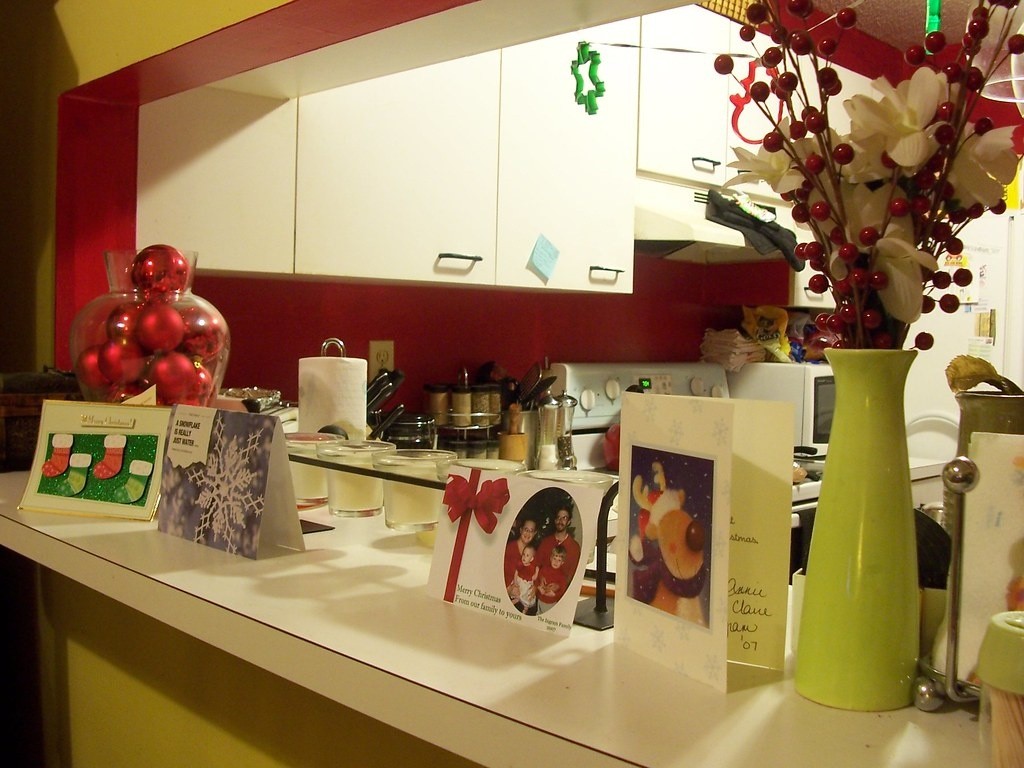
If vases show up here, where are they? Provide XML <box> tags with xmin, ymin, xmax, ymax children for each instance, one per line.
<box><xmin>795</xmin><ymin>345</ymin><xmax>924</xmax><ymax>710</ymax></box>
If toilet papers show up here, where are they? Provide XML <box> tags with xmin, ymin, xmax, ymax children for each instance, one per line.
<box><xmin>297</xmin><ymin>357</ymin><xmax>368</xmax><ymax>441</ymax></box>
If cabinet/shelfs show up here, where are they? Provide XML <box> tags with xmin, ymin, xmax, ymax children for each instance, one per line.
<box><xmin>134</xmin><ymin>2</ymin><xmax>840</xmax><ymax>306</ymax></box>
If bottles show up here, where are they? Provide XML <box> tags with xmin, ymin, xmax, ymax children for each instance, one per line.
<box><xmin>554</xmin><ymin>388</ymin><xmax>578</xmax><ymax>469</ymax></box>
<box><xmin>974</xmin><ymin>608</ymin><xmax>1022</xmax><ymax>768</ymax></box>
<box><xmin>533</xmin><ymin>388</ymin><xmax>563</xmax><ymax>470</ymax></box>
<box><xmin>69</xmin><ymin>250</ymin><xmax>231</xmax><ymax>409</ymax></box>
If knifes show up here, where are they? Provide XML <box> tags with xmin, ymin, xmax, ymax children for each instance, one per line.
<box><xmin>523</xmin><ymin>375</ymin><xmax>557</xmax><ymax>404</ymax></box>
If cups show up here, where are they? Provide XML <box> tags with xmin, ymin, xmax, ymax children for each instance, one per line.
<box><xmin>316</xmin><ymin>441</ymin><xmax>396</xmax><ymax>517</ymax></box>
<box><xmin>435</xmin><ymin>458</ymin><xmax>525</xmax><ymax>483</ymax></box>
<box><xmin>517</xmin><ymin>470</ymin><xmax>614</xmax><ymax>563</ymax></box>
<box><xmin>372</xmin><ymin>450</ymin><xmax>458</xmax><ymax>531</ymax></box>
<box><xmin>285</xmin><ymin>432</ymin><xmax>345</xmax><ymax>507</ymax></box>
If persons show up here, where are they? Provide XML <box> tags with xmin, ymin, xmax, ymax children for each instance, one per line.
<box><xmin>504</xmin><ymin>505</ymin><xmax>580</xmax><ymax>615</ymax></box>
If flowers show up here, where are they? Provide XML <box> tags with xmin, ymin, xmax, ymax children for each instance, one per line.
<box><xmin>714</xmin><ymin>0</ymin><xmax>1024</xmax><ymax>356</ymax></box>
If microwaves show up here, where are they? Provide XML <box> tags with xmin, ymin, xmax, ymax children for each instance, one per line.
<box><xmin>725</xmin><ymin>363</ymin><xmax>835</xmax><ymax>458</ymax></box>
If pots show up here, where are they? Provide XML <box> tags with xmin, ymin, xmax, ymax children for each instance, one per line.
<box><xmin>384</xmin><ymin>413</ymin><xmax>437</xmax><ymax>449</ymax></box>
